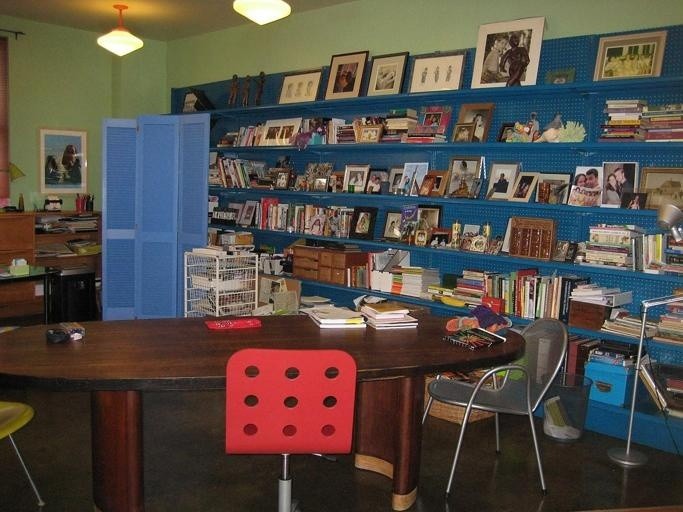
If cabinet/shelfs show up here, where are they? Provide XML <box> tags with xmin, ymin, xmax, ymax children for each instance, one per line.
<box><xmin>0</xmin><ymin>209</ymin><xmax>103</xmax><ymax>320</ymax></box>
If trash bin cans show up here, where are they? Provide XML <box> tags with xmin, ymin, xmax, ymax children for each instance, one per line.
<box><xmin>541</xmin><ymin>372</ymin><xmax>592</xmax><ymax>443</ymax></box>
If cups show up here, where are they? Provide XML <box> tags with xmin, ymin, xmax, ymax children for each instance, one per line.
<box><xmin>76</xmin><ymin>199</ymin><xmax>85</xmax><ymax>210</ymax></box>
<box><xmin>86</xmin><ymin>201</ymin><xmax>94</xmax><ymax>211</ymax></box>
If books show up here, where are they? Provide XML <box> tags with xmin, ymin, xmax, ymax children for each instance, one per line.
<box><xmin>34</xmin><ymin>213</ymin><xmax>103</xmax><ymax>298</ymax></box>
<box><xmin>187</xmin><ymin>99</ymin><xmax>682</xmax><ymax>420</ymax></box>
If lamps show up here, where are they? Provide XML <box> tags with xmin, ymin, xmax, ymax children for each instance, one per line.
<box><xmin>96</xmin><ymin>4</ymin><xmax>145</xmax><ymax>57</ymax></box>
<box><xmin>232</xmin><ymin>0</ymin><xmax>293</xmax><ymax>26</ymax></box>
<box><xmin>0</xmin><ymin>161</ymin><xmax>26</xmax><ymax>214</ymax></box>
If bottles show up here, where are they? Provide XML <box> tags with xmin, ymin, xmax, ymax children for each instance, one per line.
<box><xmin>18</xmin><ymin>193</ymin><xmax>24</xmax><ymax>211</ymax></box>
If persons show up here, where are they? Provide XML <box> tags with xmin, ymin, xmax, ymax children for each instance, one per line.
<box><xmin>602</xmin><ymin>51</ymin><xmax>654</xmax><ymax>77</ymax></box>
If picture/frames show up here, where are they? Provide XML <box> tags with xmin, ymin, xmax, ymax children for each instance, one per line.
<box><xmin>468</xmin><ymin>14</ymin><xmax>547</xmax><ymax>88</ymax></box>
<box><xmin>38</xmin><ymin>128</ymin><xmax>88</xmax><ymax>195</ymax></box>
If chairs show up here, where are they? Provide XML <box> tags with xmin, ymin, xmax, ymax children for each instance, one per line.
<box><xmin>421</xmin><ymin>317</ymin><xmax>569</xmax><ymax>501</ymax></box>
<box><xmin>222</xmin><ymin>348</ymin><xmax>359</xmax><ymax>512</ymax></box>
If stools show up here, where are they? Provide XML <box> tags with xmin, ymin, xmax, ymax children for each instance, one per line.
<box><xmin>0</xmin><ymin>400</ymin><xmax>49</xmax><ymax>507</ymax></box>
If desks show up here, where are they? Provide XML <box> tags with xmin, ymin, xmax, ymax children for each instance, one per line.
<box><xmin>0</xmin><ymin>310</ymin><xmax>529</xmax><ymax>512</ymax></box>
<box><xmin>1</xmin><ymin>264</ymin><xmax>63</xmax><ymax>325</ymax></box>
